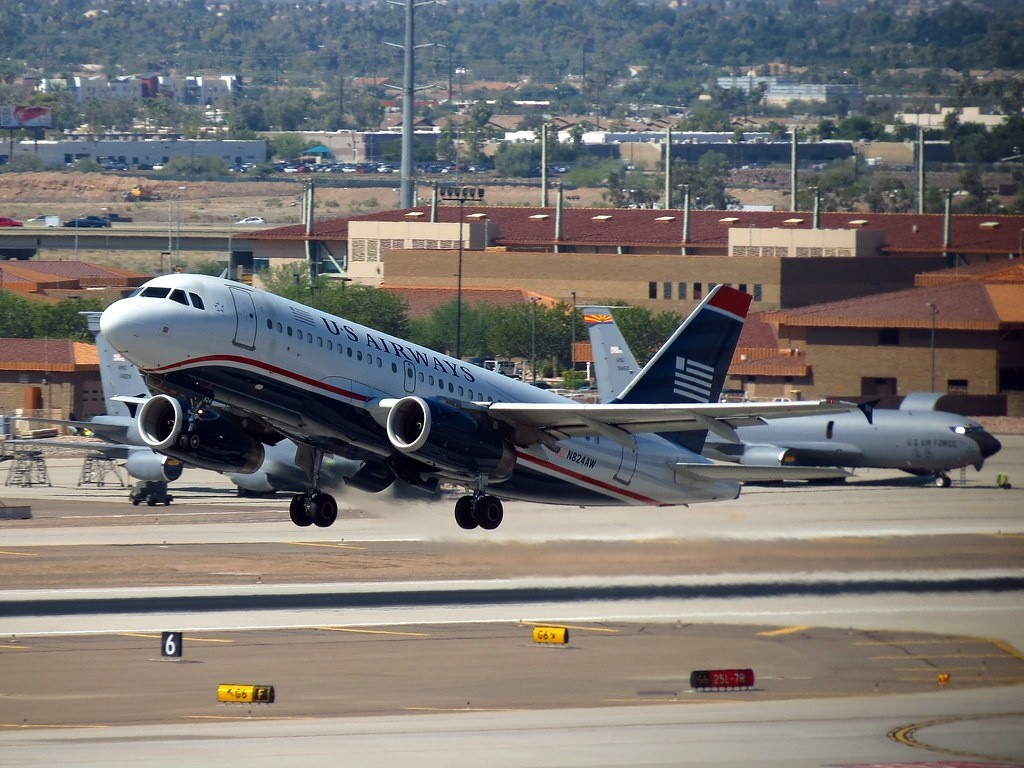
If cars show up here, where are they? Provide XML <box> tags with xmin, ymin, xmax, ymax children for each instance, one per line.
<box><xmin>27</xmin><ymin>214</ymin><xmax>53</xmax><ymax>222</ymax></box>
<box><xmin>61</xmin><ymin>214</ymin><xmax>133</xmax><ymax>227</ymax></box>
<box><xmin>234</xmin><ymin>216</ymin><xmax>267</xmax><ymax>225</ymax></box>
<box><xmin>0</xmin><ymin>216</ymin><xmax>23</xmax><ymax>227</ymax></box>
<box><xmin>95</xmin><ymin>161</ymin><xmax>575</xmax><ymax>174</ymax></box>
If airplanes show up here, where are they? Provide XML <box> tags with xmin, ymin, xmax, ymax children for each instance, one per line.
<box><xmin>574</xmin><ymin>302</ymin><xmax>1004</xmax><ymax>491</ymax></box>
<box><xmin>1</xmin><ymin>260</ymin><xmax>861</xmax><ymax>530</ymax></box>
